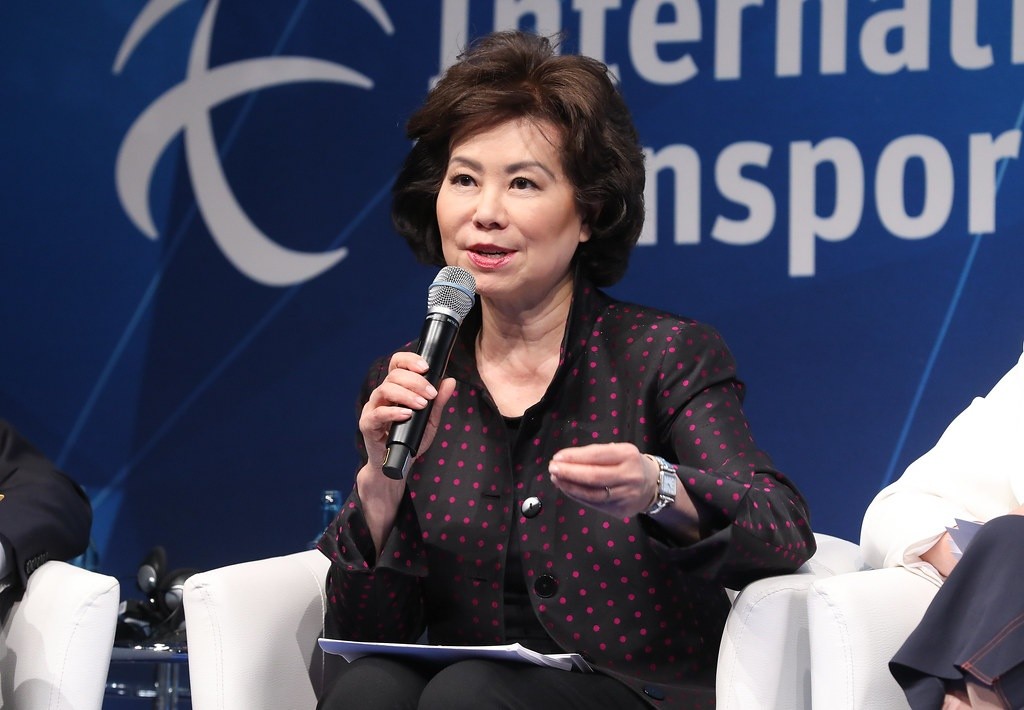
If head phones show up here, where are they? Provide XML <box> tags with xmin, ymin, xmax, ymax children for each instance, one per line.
<box><xmin>131</xmin><ymin>544</ymin><xmax>202</xmax><ymax>640</ymax></box>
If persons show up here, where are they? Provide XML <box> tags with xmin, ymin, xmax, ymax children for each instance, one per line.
<box><xmin>856</xmin><ymin>350</ymin><xmax>1024</xmax><ymax>710</ymax></box>
<box><xmin>0</xmin><ymin>417</ymin><xmax>92</xmax><ymax>594</ymax></box>
<box><xmin>315</xmin><ymin>35</ymin><xmax>818</xmax><ymax>710</ymax></box>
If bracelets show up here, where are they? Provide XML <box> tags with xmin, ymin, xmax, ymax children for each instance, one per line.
<box><xmin>645</xmin><ymin>452</ymin><xmax>660</xmax><ymax>508</ymax></box>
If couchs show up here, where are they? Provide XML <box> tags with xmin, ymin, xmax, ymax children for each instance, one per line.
<box><xmin>0</xmin><ymin>532</ymin><xmax>945</xmax><ymax>710</ymax></box>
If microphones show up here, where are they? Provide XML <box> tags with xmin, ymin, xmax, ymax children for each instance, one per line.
<box><xmin>380</xmin><ymin>266</ymin><xmax>478</xmax><ymax>481</ymax></box>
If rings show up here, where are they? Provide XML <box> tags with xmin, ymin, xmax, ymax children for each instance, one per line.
<box><xmin>605</xmin><ymin>486</ymin><xmax>612</xmax><ymax>502</ymax></box>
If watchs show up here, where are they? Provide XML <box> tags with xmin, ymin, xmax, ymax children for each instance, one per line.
<box><xmin>649</xmin><ymin>455</ymin><xmax>676</xmax><ymax>515</ymax></box>
